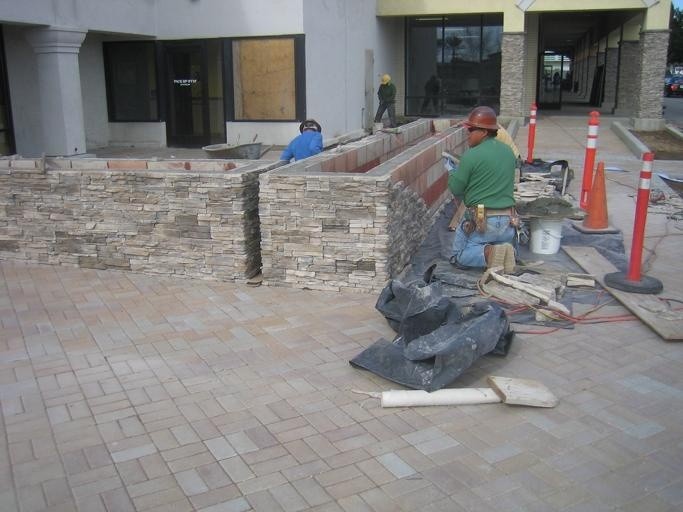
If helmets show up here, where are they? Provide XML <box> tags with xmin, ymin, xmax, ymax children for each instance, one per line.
<box><xmin>460</xmin><ymin>105</ymin><xmax>499</xmax><ymax>131</ymax></box>
<box><xmin>380</xmin><ymin>74</ymin><xmax>392</xmax><ymax>85</ymax></box>
<box><xmin>299</xmin><ymin>118</ymin><xmax>321</xmax><ymax>133</ymax></box>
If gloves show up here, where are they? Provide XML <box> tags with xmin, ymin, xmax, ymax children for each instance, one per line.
<box><xmin>444</xmin><ymin>156</ymin><xmax>456</xmax><ymax>172</ymax></box>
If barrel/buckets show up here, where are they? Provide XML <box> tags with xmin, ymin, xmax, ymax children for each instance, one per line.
<box><xmin>372</xmin><ymin>122</ymin><xmax>383</xmax><ymax>135</ymax></box>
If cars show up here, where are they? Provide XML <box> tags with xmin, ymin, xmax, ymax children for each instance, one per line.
<box><xmin>664</xmin><ymin>73</ymin><xmax>682</xmax><ymax>99</ymax></box>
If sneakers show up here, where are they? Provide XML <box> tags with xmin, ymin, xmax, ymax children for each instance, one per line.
<box><xmin>483</xmin><ymin>242</ymin><xmax>517</xmax><ymax>275</ymax></box>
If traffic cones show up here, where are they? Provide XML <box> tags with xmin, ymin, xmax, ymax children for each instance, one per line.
<box><xmin>571</xmin><ymin>161</ymin><xmax>621</xmax><ymax>234</ymax></box>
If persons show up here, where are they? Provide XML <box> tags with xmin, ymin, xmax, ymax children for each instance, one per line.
<box><xmin>444</xmin><ymin>105</ymin><xmax>517</xmax><ymax>275</ymax></box>
<box><xmin>279</xmin><ymin>119</ymin><xmax>323</xmax><ymax>161</ymax></box>
<box><xmin>420</xmin><ymin>76</ymin><xmax>440</xmax><ymax>114</ymax></box>
<box><xmin>374</xmin><ymin>73</ymin><xmax>397</xmax><ymax>128</ymax></box>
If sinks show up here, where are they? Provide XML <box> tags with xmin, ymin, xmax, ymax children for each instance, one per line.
<box><xmin>529</xmin><ymin>218</ymin><xmax>565</xmax><ymax>255</ymax></box>
